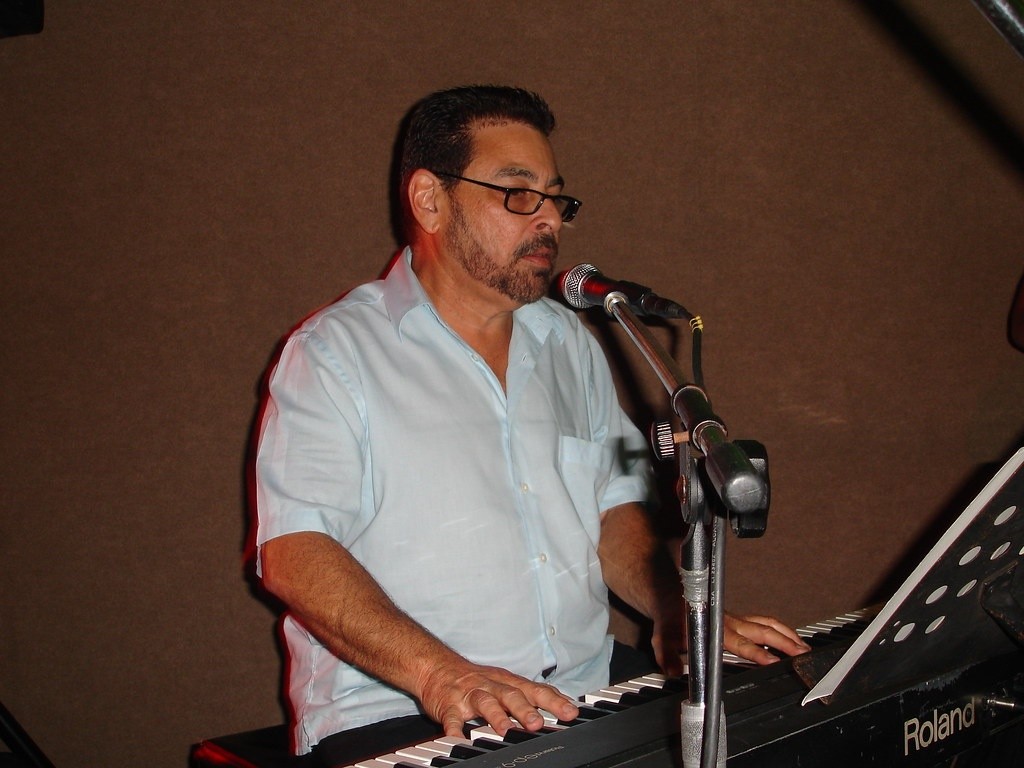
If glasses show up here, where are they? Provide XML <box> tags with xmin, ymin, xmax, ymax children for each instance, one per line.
<box><xmin>431</xmin><ymin>169</ymin><xmax>582</xmax><ymax>223</ymax></box>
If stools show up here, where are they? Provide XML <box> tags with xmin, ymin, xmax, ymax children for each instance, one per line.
<box><xmin>189</xmin><ymin>725</ymin><xmax>288</xmax><ymax>768</ymax></box>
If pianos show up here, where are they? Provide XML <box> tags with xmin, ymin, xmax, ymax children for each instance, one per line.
<box><xmin>332</xmin><ymin>601</ymin><xmax>1023</xmax><ymax>767</ymax></box>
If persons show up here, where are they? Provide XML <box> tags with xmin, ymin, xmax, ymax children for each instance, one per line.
<box><xmin>253</xmin><ymin>83</ymin><xmax>813</xmax><ymax>768</ymax></box>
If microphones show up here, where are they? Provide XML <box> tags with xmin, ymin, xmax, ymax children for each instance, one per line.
<box><xmin>561</xmin><ymin>262</ymin><xmax>686</xmax><ymax>319</ymax></box>
